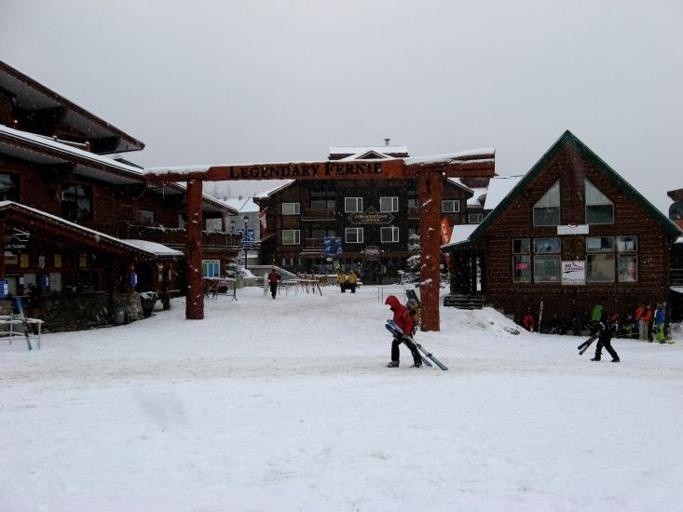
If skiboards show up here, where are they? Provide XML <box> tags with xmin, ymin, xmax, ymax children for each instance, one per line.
<box><xmin>297</xmin><ymin>270</ymin><xmax>323</xmax><ymax>296</ymax></box>
<box><xmin>578</xmin><ymin>317</ymin><xmax>621</xmax><ymax>355</ymax></box>
<box><xmin>386</xmin><ymin>320</ymin><xmax>448</xmax><ymax>371</ymax></box>
<box><xmin>15</xmin><ymin>296</ymin><xmax>33</xmax><ymax>351</ymax></box>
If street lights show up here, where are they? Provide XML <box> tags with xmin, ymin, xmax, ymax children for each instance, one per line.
<box><xmin>243</xmin><ymin>217</ymin><xmax>249</xmax><ymax>268</ymax></box>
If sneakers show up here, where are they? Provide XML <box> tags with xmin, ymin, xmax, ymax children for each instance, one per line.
<box><xmin>590</xmin><ymin>355</ymin><xmax>621</xmax><ymax>362</ymax></box>
<box><xmin>387</xmin><ymin>360</ymin><xmax>422</xmax><ymax>368</ymax></box>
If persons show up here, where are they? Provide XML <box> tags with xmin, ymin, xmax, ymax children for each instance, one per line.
<box><xmin>522</xmin><ymin>298</ymin><xmax>672</xmax><ymax>344</ymax></box>
<box><xmin>406</xmin><ymin>290</ymin><xmax>420</xmax><ymax>326</ymax></box>
<box><xmin>349</xmin><ymin>269</ymin><xmax>357</xmax><ymax>293</ymax></box>
<box><xmin>338</xmin><ymin>271</ymin><xmax>346</xmax><ymax>292</ymax></box>
<box><xmin>268</xmin><ymin>269</ymin><xmax>280</xmax><ymax>299</ymax></box>
<box><xmin>589</xmin><ymin>319</ymin><xmax>620</xmax><ymax>362</ymax></box>
<box><xmin>385</xmin><ymin>295</ymin><xmax>422</xmax><ymax>368</ymax></box>
